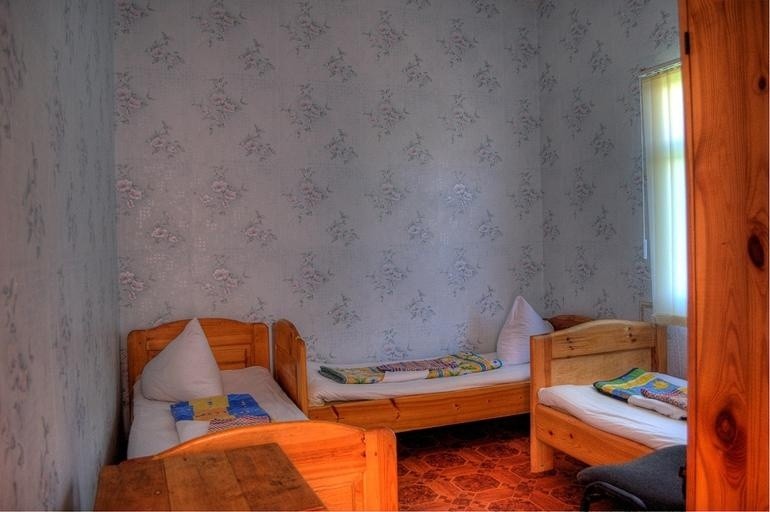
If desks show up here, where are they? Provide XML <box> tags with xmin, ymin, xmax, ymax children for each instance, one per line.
<box><xmin>94</xmin><ymin>443</ymin><xmax>331</xmax><ymax>512</ymax></box>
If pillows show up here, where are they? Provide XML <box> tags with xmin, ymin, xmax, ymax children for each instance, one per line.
<box><xmin>496</xmin><ymin>295</ymin><xmax>555</xmax><ymax>364</ymax></box>
<box><xmin>139</xmin><ymin>319</ymin><xmax>224</xmax><ymax>403</ymax></box>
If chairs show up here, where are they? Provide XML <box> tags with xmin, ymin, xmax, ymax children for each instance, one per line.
<box><xmin>573</xmin><ymin>445</ymin><xmax>687</xmax><ymax>511</ymax></box>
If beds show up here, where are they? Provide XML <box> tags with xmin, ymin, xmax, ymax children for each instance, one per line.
<box><xmin>529</xmin><ymin>318</ymin><xmax>690</xmax><ymax>474</ymax></box>
<box><xmin>272</xmin><ymin>316</ymin><xmax>599</xmax><ymax>433</ymax></box>
<box><xmin>126</xmin><ymin>318</ymin><xmax>399</xmax><ymax>511</ymax></box>
